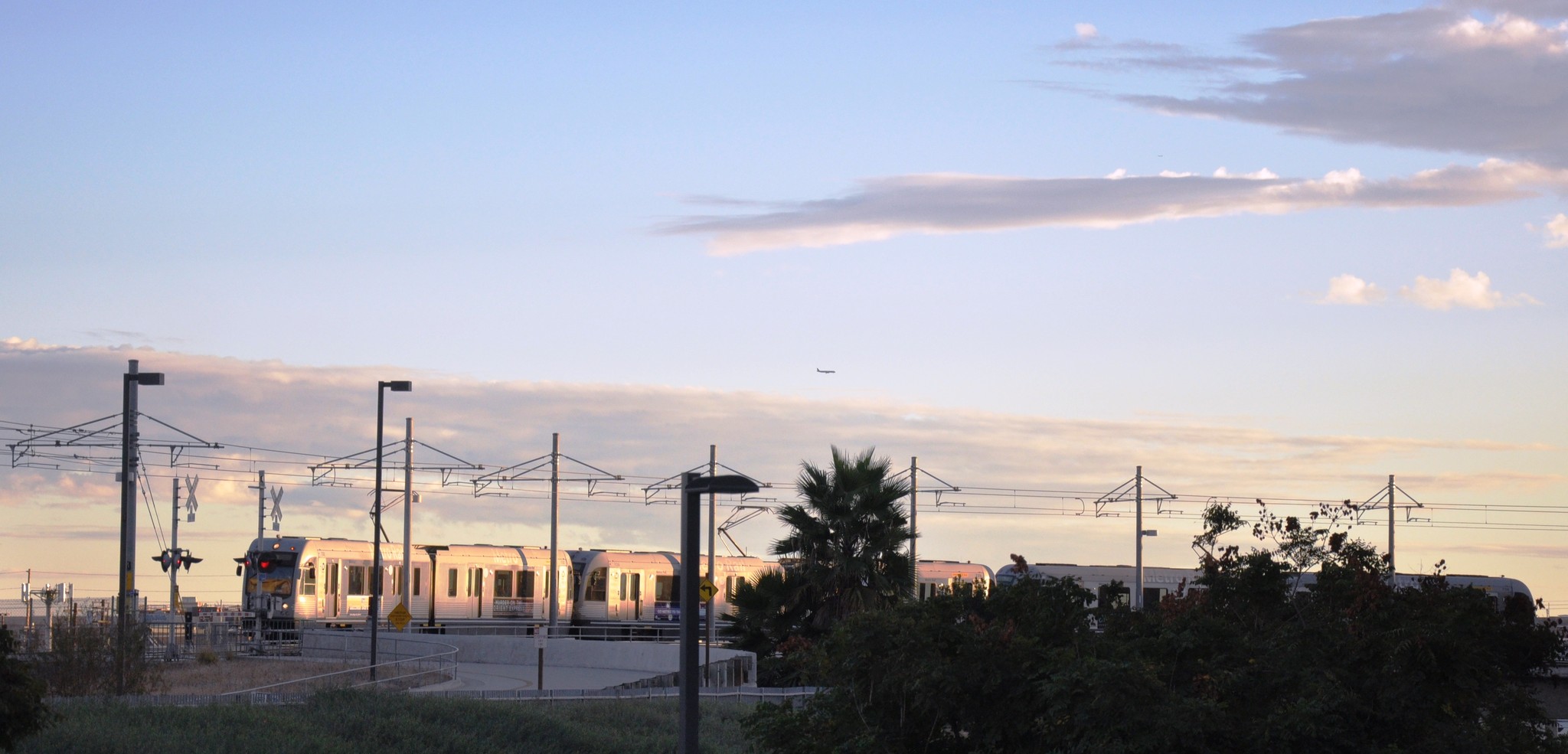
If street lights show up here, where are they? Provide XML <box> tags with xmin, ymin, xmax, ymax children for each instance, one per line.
<box><xmin>369</xmin><ymin>380</ymin><xmax>412</xmax><ymax>684</ymax></box>
<box><xmin>1135</xmin><ymin>529</ymin><xmax>1157</xmax><ymax>612</ymax></box>
<box><xmin>677</xmin><ymin>473</ymin><xmax>760</xmax><ymax>754</ymax></box>
<box><xmin>116</xmin><ymin>372</ymin><xmax>165</xmax><ymax>697</ymax></box>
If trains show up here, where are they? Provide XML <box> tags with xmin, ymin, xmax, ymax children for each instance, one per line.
<box><xmin>232</xmin><ymin>536</ymin><xmax>1535</xmax><ymax>648</ymax></box>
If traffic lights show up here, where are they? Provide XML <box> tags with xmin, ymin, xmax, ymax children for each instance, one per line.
<box><xmin>241</xmin><ymin>557</ymin><xmax>252</xmax><ymax>568</ymax></box>
<box><xmin>172</xmin><ymin>552</ymin><xmax>182</xmax><ymax>569</ymax></box>
<box><xmin>259</xmin><ymin>551</ymin><xmax>278</xmax><ymax>574</ymax></box>
<box><xmin>183</xmin><ymin>555</ymin><xmax>192</xmax><ymax>570</ymax></box>
<box><xmin>160</xmin><ymin>551</ymin><xmax>169</xmax><ymax>573</ymax></box>
